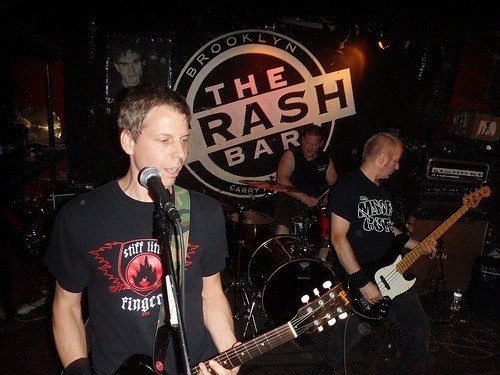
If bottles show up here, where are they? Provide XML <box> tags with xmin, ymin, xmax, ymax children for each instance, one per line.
<box><xmin>450</xmin><ymin>288</ymin><xmax>463</xmax><ymax>312</ymax></box>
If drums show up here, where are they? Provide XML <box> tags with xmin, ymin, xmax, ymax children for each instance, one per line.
<box><xmin>319</xmin><ymin>204</ymin><xmax>331</xmax><ymax>240</ymax></box>
<box><xmin>248</xmin><ymin>234</ymin><xmax>336</xmax><ymax>327</ymax></box>
<box><xmin>289</xmin><ymin>216</ymin><xmax>317</xmax><ymax>237</ymax></box>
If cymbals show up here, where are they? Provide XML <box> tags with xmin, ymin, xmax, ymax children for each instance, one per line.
<box><xmin>239</xmin><ymin>180</ymin><xmax>302</xmax><ymax>192</ymax></box>
<box><xmin>229</xmin><ymin>210</ymin><xmax>274</xmax><ymax>224</ymax></box>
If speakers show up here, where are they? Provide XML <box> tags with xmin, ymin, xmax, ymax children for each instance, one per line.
<box><xmin>466</xmin><ymin>255</ymin><xmax>500</xmax><ymax>320</ymax></box>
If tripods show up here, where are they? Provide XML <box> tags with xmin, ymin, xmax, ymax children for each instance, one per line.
<box><xmin>423</xmin><ymin>238</ymin><xmax>446</xmax><ymax>292</ymax></box>
<box><xmin>225</xmin><ymin>194</ymin><xmax>269</xmax><ymax>321</ymax></box>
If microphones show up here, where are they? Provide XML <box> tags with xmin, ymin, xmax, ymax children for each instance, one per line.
<box><xmin>138</xmin><ymin>166</ymin><xmax>181</xmax><ymax>226</ymax></box>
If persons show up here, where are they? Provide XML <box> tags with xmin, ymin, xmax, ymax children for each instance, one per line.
<box><xmin>315</xmin><ymin>133</ymin><xmax>438</xmax><ymax>375</ymax></box>
<box><xmin>45</xmin><ymin>83</ymin><xmax>245</xmax><ymax>375</ymax></box>
<box><xmin>105</xmin><ymin>42</ymin><xmax>146</xmax><ymax>115</ymax></box>
<box><xmin>274</xmin><ymin>125</ymin><xmax>337</xmax><ymax>265</ymax></box>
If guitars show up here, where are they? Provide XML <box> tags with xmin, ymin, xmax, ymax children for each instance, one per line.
<box><xmin>113</xmin><ymin>280</ymin><xmax>355</xmax><ymax>375</ymax></box>
<box><xmin>347</xmin><ymin>185</ymin><xmax>491</xmax><ymax>321</ymax></box>
